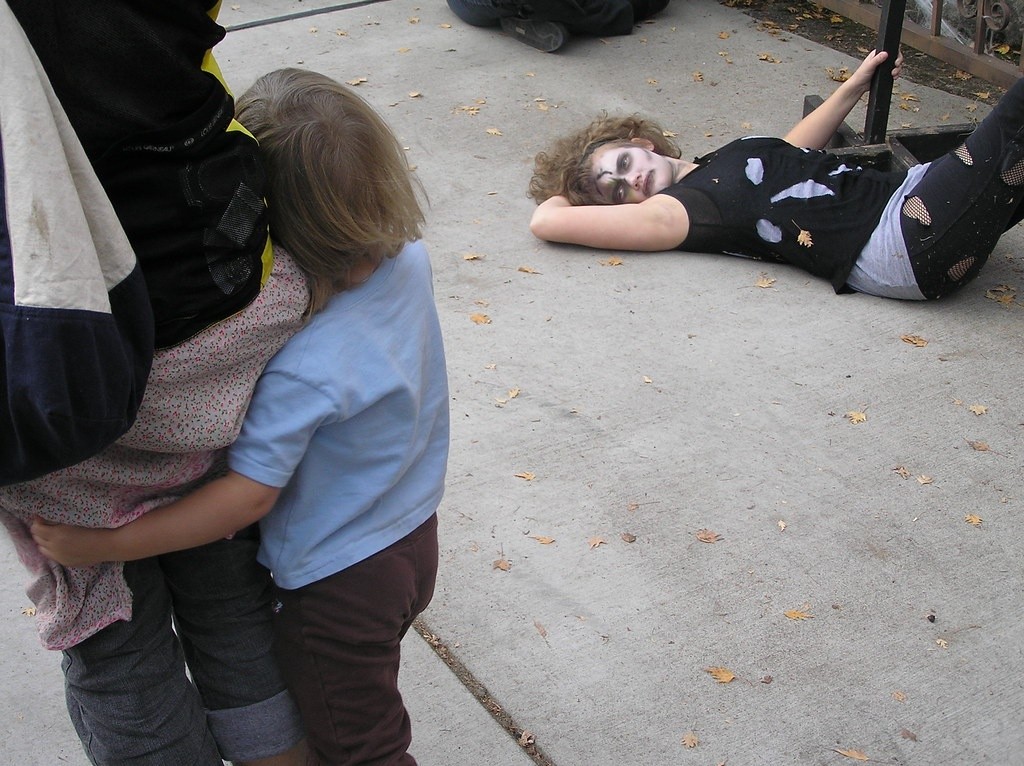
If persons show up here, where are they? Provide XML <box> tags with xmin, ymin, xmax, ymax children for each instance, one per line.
<box><xmin>526</xmin><ymin>49</ymin><xmax>1024</xmax><ymax>301</ymax></box>
<box><xmin>31</xmin><ymin>67</ymin><xmax>448</xmax><ymax>766</ymax></box>
<box><xmin>0</xmin><ymin>0</ymin><xmax>316</xmax><ymax>766</ymax></box>
<box><xmin>447</xmin><ymin>0</ymin><xmax>670</xmax><ymax>53</ymax></box>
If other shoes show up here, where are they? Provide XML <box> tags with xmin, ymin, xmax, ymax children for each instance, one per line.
<box><xmin>501</xmin><ymin>15</ymin><xmax>569</xmax><ymax>51</ymax></box>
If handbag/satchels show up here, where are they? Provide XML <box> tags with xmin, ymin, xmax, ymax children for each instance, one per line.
<box><xmin>0</xmin><ymin>0</ymin><xmax>154</xmax><ymax>488</ymax></box>
